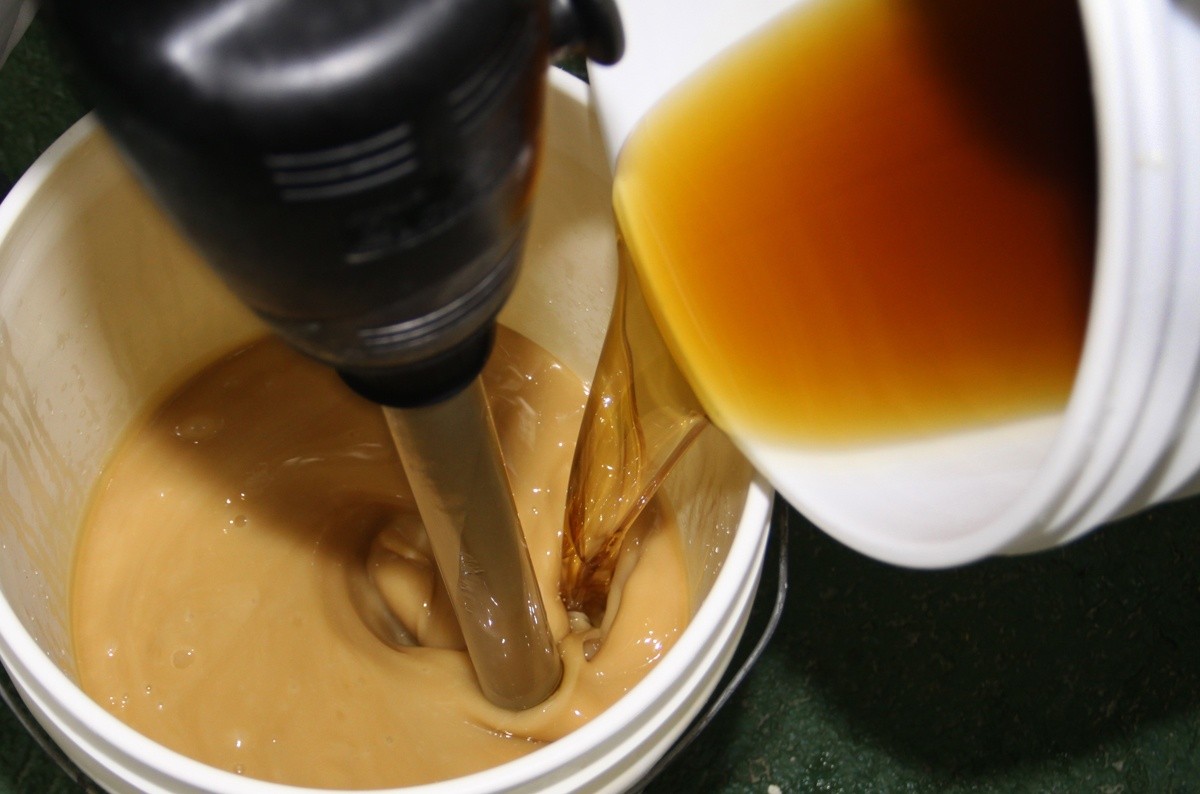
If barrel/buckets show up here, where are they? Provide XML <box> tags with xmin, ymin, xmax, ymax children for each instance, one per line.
<box><xmin>0</xmin><ymin>59</ymin><xmax>791</xmax><ymax>794</ymax></box>
<box><xmin>586</xmin><ymin>1</ymin><xmax>1199</xmax><ymax>567</ymax></box>
